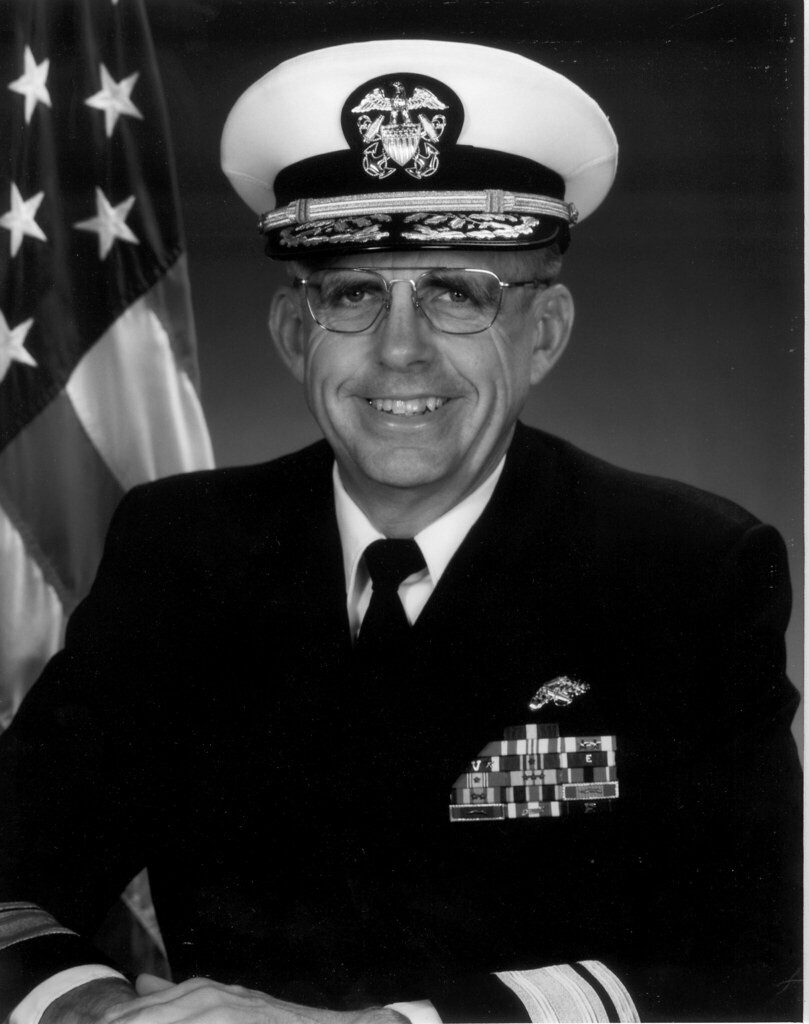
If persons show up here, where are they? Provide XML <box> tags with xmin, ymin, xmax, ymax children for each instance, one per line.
<box><xmin>4</xmin><ymin>31</ymin><xmax>801</xmax><ymax>1022</ymax></box>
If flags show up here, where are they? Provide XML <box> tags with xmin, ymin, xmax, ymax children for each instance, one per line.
<box><xmin>1</xmin><ymin>1</ymin><xmax>225</xmax><ymax>743</ymax></box>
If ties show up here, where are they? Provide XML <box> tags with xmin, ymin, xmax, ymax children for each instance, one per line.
<box><xmin>352</xmin><ymin>538</ymin><xmax>424</xmax><ymax>676</ymax></box>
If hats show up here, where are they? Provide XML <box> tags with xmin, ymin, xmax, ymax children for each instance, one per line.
<box><xmin>220</xmin><ymin>39</ymin><xmax>622</xmax><ymax>258</ymax></box>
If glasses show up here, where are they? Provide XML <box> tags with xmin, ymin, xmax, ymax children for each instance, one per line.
<box><xmin>281</xmin><ymin>266</ymin><xmax>562</xmax><ymax>336</ymax></box>
